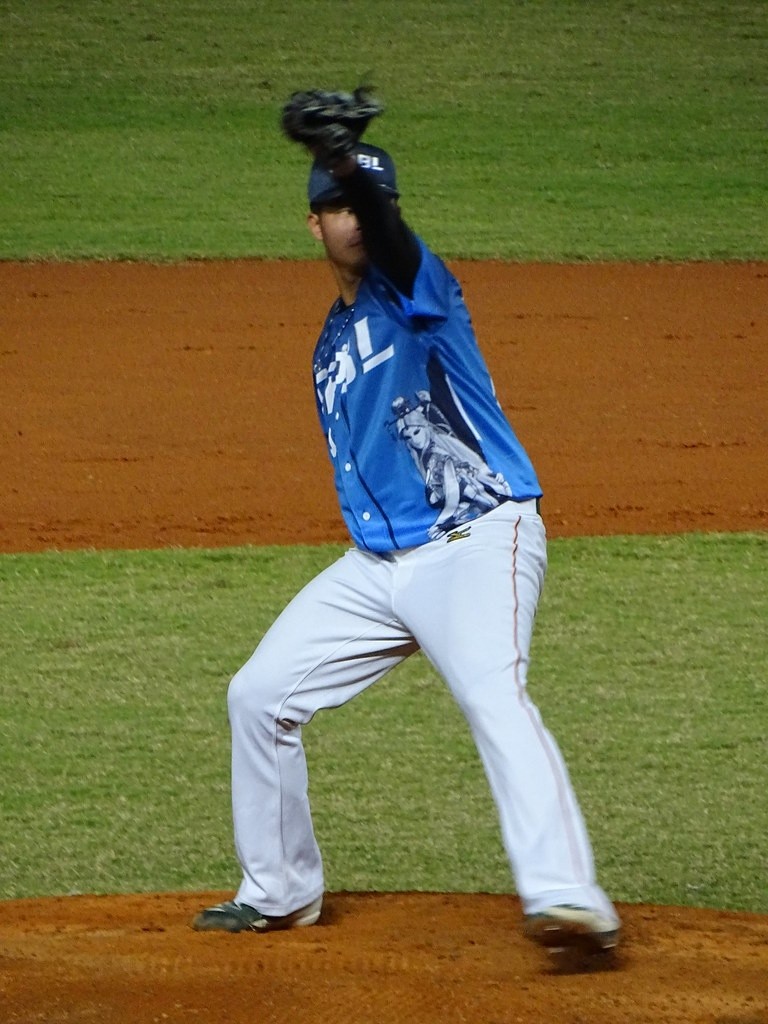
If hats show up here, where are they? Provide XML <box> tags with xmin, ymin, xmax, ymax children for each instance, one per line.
<box><xmin>307</xmin><ymin>143</ymin><xmax>399</xmax><ymax>210</ymax></box>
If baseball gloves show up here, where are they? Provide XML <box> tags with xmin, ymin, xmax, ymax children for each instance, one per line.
<box><xmin>281</xmin><ymin>83</ymin><xmax>386</xmax><ymax>174</ymax></box>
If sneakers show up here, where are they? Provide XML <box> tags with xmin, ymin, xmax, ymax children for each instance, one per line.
<box><xmin>190</xmin><ymin>900</ymin><xmax>325</xmax><ymax>933</ymax></box>
<box><xmin>525</xmin><ymin>904</ymin><xmax>621</xmax><ymax>972</ymax></box>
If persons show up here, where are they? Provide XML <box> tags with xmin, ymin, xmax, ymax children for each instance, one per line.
<box><xmin>192</xmin><ymin>87</ymin><xmax>622</xmax><ymax>972</ymax></box>
